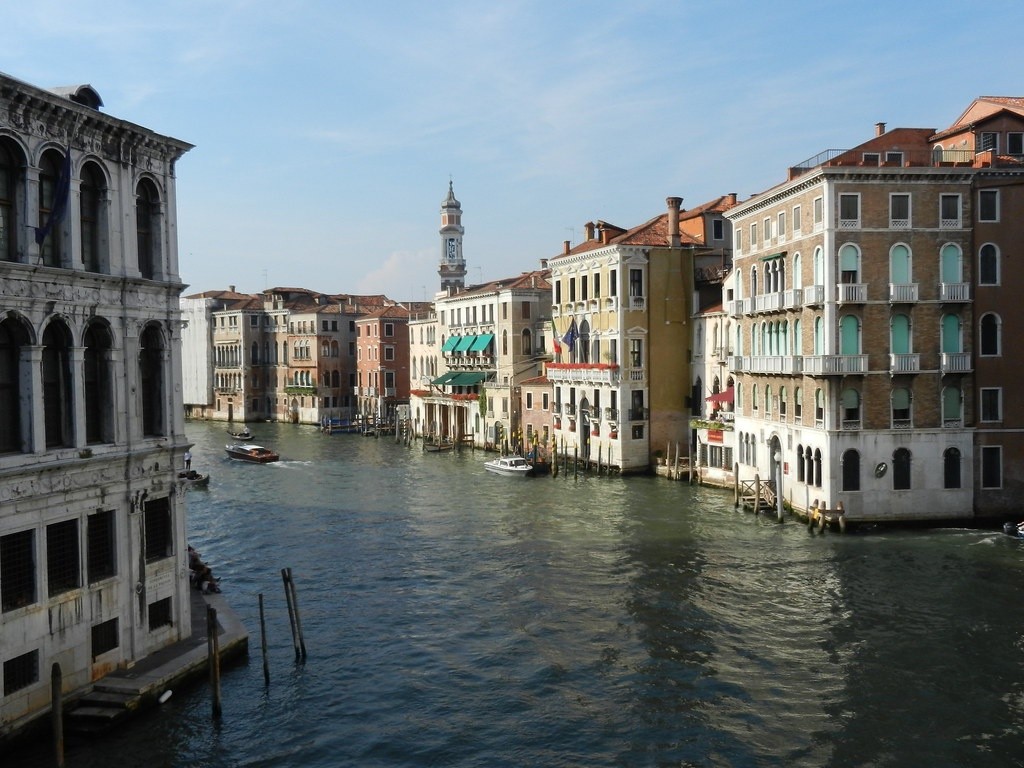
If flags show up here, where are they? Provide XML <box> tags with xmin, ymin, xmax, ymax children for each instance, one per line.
<box><xmin>551</xmin><ymin>320</ymin><xmax>579</xmax><ymax>353</ymax></box>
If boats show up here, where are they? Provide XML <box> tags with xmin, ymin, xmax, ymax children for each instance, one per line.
<box><xmin>226</xmin><ymin>430</ymin><xmax>255</xmax><ymax>441</ymax></box>
<box><xmin>187</xmin><ymin>474</ymin><xmax>210</xmax><ymax>485</ymax></box>
<box><xmin>225</xmin><ymin>443</ymin><xmax>279</xmax><ymax>463</ymax></box>
<box><xmin>484</xmin><ymin>454</ymin><xmax>533</xmax><ymax>478</ymax></box>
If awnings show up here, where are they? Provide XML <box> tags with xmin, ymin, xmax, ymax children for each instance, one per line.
<box><xmin>441</xmin><ymin>334</ymin><xmax>494</xmax><ymax>351</ymax></box>
<box><xmin>432</xmin><ymin>372</ymin><xmax>493</xmax><ymax>386</ymax></box>
<box><xmin>704</xmin><ymin>387</ymin><xmax>735</xmax><ymax>404</ymax></box>
